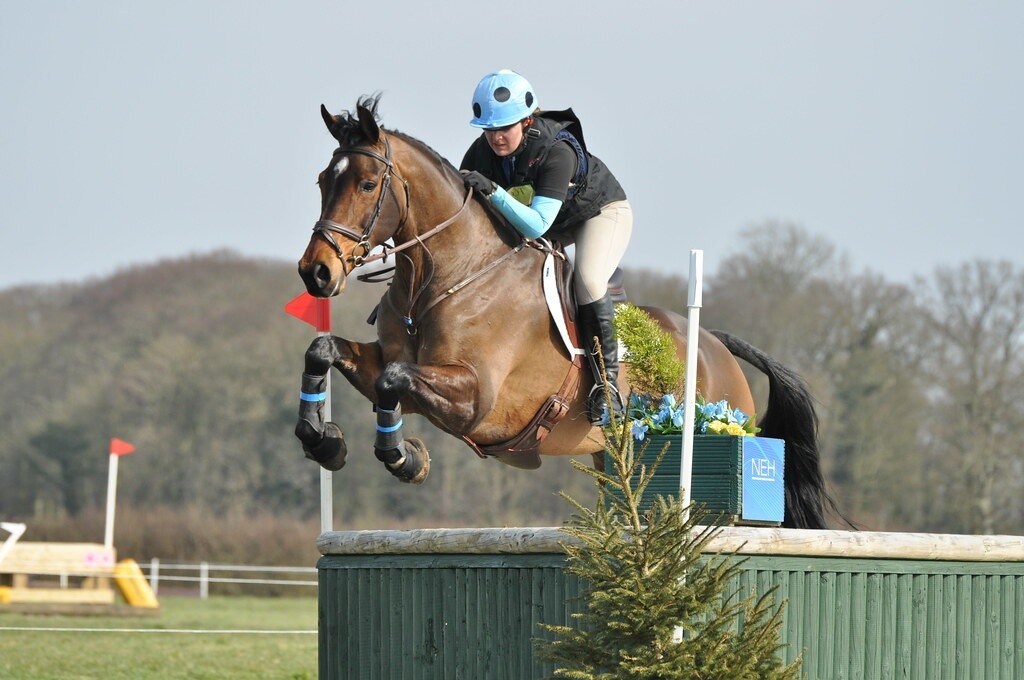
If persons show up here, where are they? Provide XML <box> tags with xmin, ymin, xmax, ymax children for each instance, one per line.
<box><xmin>459</xmin><ymin>69</ymin><xmax>633</xmax><ymax>423</ymax></box>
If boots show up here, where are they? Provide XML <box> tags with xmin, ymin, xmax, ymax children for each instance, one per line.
<box><xmin>577</xmin><ymin>289</ymin><xmax>622</xmax><ymax>418</ymax></box>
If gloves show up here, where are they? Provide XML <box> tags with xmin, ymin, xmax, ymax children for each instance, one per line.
<box><xmin>464</xmin><ymin>170</ymin><xmax>498</xmax><ymax>195</ymax></box>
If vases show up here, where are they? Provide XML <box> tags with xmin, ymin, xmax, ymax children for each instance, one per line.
<box><xmin>605</xmin><ymin>436</ymin><xmax>786</xmax><ymax>527</ymax></box>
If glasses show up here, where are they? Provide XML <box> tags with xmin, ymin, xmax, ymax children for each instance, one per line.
<box><xmin>483</xmin><ymin>120</ymin><xmax>523</xmax><ymax>131</ymax></box>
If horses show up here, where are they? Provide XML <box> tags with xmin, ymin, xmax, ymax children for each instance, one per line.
<box><xmin>293</xmin><ymin>90</ymin><xmax>864</xmax><ymax>530</ymax></box>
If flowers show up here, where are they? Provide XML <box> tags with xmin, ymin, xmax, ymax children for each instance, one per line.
<box><xmin>593</xmin><ymin>301</ymin><xmax>762</xmax><ymax>439</ymax></box>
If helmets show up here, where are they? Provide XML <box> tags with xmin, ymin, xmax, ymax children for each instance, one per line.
<box><xmin>469</xmin><ymin>69</ymin><xmax>539</xmax><ymax>129</ymax></box>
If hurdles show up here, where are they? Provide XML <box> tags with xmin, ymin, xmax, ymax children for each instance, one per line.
<box><xmin>0</xmin><ymin>453</ymin><xmax>121</xmax><ymax>605</ymax></box>
<box><xmin>314</xmin><ymin>246</ymin><xmax>1023</xmax><ymax>680</ymax></box>
